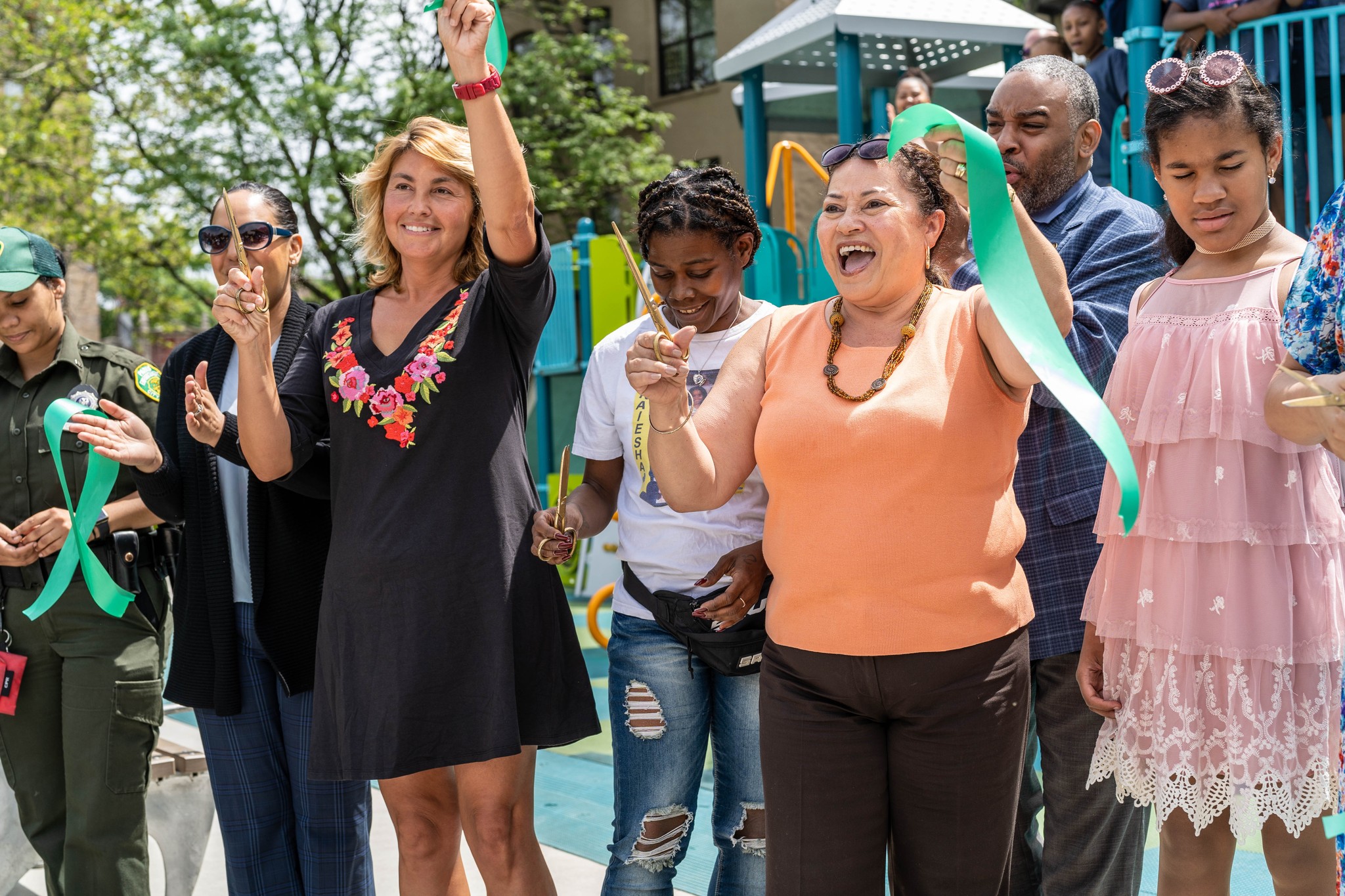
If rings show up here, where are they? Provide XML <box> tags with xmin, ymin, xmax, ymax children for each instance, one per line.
<box><xmin>194</xmin><ymin>403</ymin><xmax>203</xmax><ymax>417</ymax></box>
<box><xmin>953</xmin><ymin>162</ymin><xmax>966</xmax><ymax>178</ymax></box>
<box><xmin>738</xmin><ymin>597</ymin><xmax>746</xmax><ymax>609</ymax></box>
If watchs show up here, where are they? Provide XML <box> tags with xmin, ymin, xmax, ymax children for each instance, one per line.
<box><xmin>451</xmin><ymin>62</ymin><xmax>503</xmax><ymax>102</ymax></box>
<box><xmin>95</xmin><ymin>505</ymin><xmax>111</xmax><ymax>538</ymax></box>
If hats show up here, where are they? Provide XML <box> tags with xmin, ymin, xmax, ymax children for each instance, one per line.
<box><xmin>0</xmin><ymin>226</ymin><xmax>64</xmax><ymax>292</ymax></box>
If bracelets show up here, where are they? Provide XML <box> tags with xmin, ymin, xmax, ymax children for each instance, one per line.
<box><xmin>646</xmin><ymin>388</ymin><xmax>697</xmax><ymax>434</ymax></box>
<box><xmin>1010</xmin><ymin>184</ymin><xmax>1015</xmax><ymax>202</ymax></box>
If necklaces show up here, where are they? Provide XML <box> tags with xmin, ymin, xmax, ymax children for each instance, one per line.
<box><xmin>821</xmin><ymin>280</ymin><xmax>934</xmax><ymax>404</ymax></box>
<box><xmin>675</xmin><ymin>290</ymin><xmax>743</xmax><ymax>388</ymax></box>
<box><xmin>1195</xmin><ymin>212</ymin><xmax>1276</xmax><ymax>256</ymax></box>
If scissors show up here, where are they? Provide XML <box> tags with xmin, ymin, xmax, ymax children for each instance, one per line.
<box><xmin>611</xmin><ymin>221</ymin><xmax>692</xmax><ymax>368</ymax></box>
<box><xmin>537</xmin><ymin>444</ymin><xmax>576</xmax><ymax>561</ymax></box>
<box><xmin>222</xmin><ymin>188</ymin><xmax>272</xmax><ymax>312</ymax></box>
<box><xmin>1275</xmin><ymin>361</ymin><xmax>1345</xmax><ymax>408</ymax></box>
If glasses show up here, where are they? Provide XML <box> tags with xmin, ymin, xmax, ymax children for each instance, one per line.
<box><xmin>196</xmin><ymin>221</ymin><xmax>294</xmax><ymax>254</ymax></box>
<box><xmin>820</xmin><ymin>137</ymin><xmax>942</xmax><ymax>203</ymax></box>
<box><xmin>1019</xmin><ymin>37</ymin><xmax>1047</xmax><ymax>56</ymax></box>
<box><xmin>1145</xmin><ymin>48</ymin><xmax>1263</xmax><ymax>99</ymax></box>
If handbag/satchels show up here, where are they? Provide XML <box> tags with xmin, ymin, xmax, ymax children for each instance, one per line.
<box><xmin>620</xmin><ymin>560</ymin><xmax>765</xmax><ymax>676</ymax></box>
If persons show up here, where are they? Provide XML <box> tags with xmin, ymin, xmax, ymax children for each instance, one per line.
<box><xmin>1018</xmin><ymin>27</ymin><xmax>1068</xmax><ymax>62</ymax></box>
<box><xmin>67</xmin><ymin>182</ymin><xmax>377</xmax><ymax>896</ymax></box>
<box><xmin>1160</xmin><ymin>0</ymin><xmax>1306</xmax><ymax>233</ymax></box>
<box><xmin>951</xmin><ymin>54</ymin><xmax>1174</xmax><ymax>895</ymax></box>
<box><xmin>0</xmin><ymin>223</ymin><xmax>180</xmax><ymax>896</ymax></box>
<box><xmin>885</xmin><ymin>67</ymin><xmax>933</xmax><ymax>127</ymax></box>
<box><xmin>1060</xmin><ymin>1</ymin><xmax>1131</xmax><ymax>190</ymax></box>
<box><xmin>531</xmin><ymin>167</ymin><xmax>777</xmax><ymax>896</ymax></box>
<box><xmin>625</xmin><ymin>125</ymin><xmax>1071</xmax><ymax>896</ymax></box>
<box><xmin>1082</xmin><ymin>47</ymin><xmax>1345</xmax><ymax>896</ymax></box>
<box><xmin>1262</xmin><ymin>184</ymin><xmax>1345</xmax><ymax>503</ymax></box>
<box><xmin>210</xmin><ymin>0</ymin><xmax>602</xmax><ymax>896</ymax></box>
